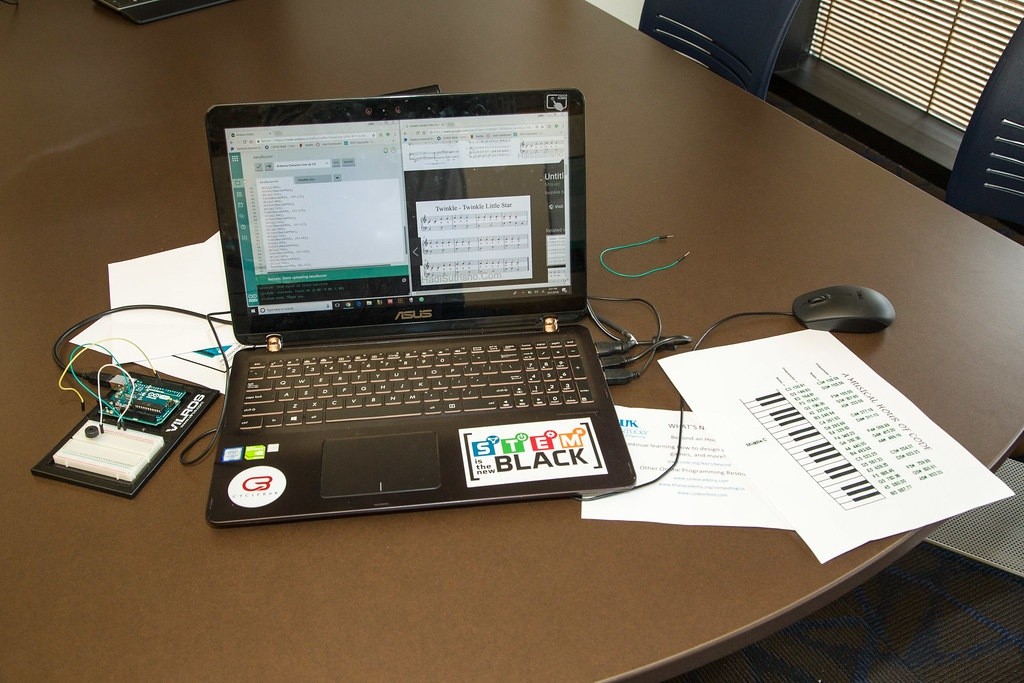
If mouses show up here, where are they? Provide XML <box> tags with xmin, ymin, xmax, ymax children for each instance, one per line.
<box><xmin>792</xmin><ymin>285</ymin><xmax>895</xmax><ymax>333</ymax></box>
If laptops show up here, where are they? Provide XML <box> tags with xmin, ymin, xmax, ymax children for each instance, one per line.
<box><xmin>206</xmin><ymin>87</ymin><xmax>637</xmax><ymax>527</ymax></box>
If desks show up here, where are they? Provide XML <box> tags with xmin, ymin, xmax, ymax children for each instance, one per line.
<box><xmin>0</xmin><ymin>0</ymin><xmax>1024</xmax><ymax>683</ymax></box>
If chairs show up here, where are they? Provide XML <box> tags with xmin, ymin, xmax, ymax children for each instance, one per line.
<box><xmin>946</xmin><ymin>18</ymin><xmax>1024</xmax><ymax>231</ymax></box>
<box><xmin>638</xmin><ymin>1</ymin><xmax>802</xmax><ymax>103</ymax></box>
